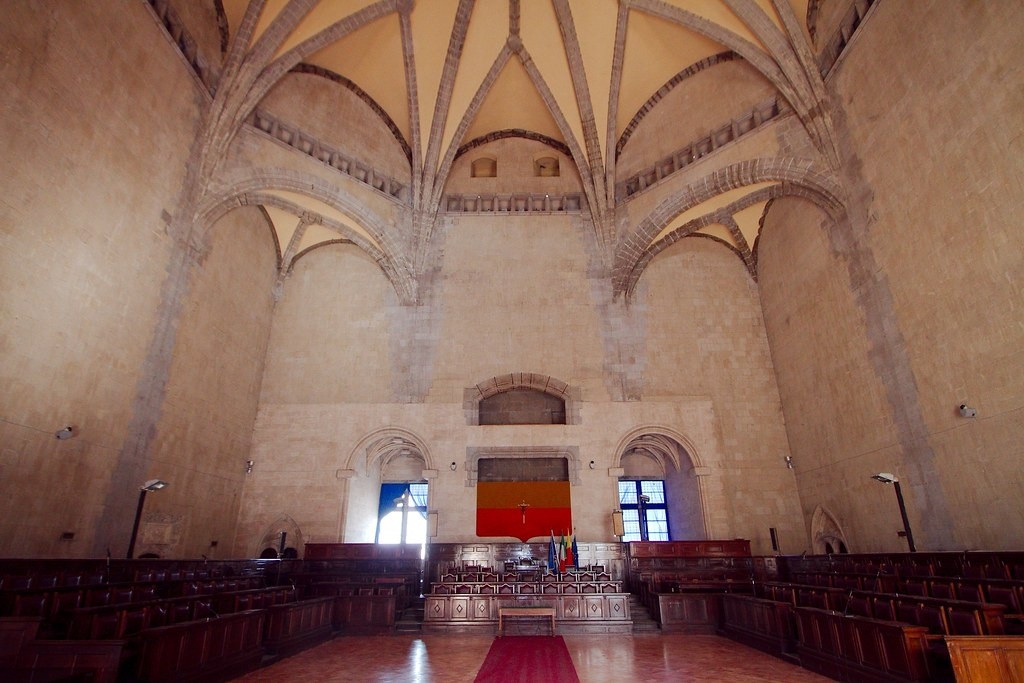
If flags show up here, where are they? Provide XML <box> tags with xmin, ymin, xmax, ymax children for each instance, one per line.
<box><xmin>548</xmin><ymin>526</ymin><xmax>581</xmax><ymax>573</ymax></box>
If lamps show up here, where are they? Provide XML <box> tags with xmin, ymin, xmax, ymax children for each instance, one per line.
<box><xmin>871</xmin><ymin>472</ymin><xmax>915</xmax><ymax>553</ymax></box>
<box><xmin>124</xmin><ymin>478</ymin><xmax>168</xmax><ymax>559</ymax></box>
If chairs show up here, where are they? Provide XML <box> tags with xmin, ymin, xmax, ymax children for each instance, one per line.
<box><xmin>425</xmin><ymin>556</ymin><xmax>627</xmax><ymax>635</ymax></box>
<box><xmin>628</xmin><ymin>538</ymin><xmax>1024</xmax><ymax>683</ymax></box>
<box><xmin>0</xmin><ymin>543</ymin><xmax>423</xmax><ymax>682</ymax></box>
